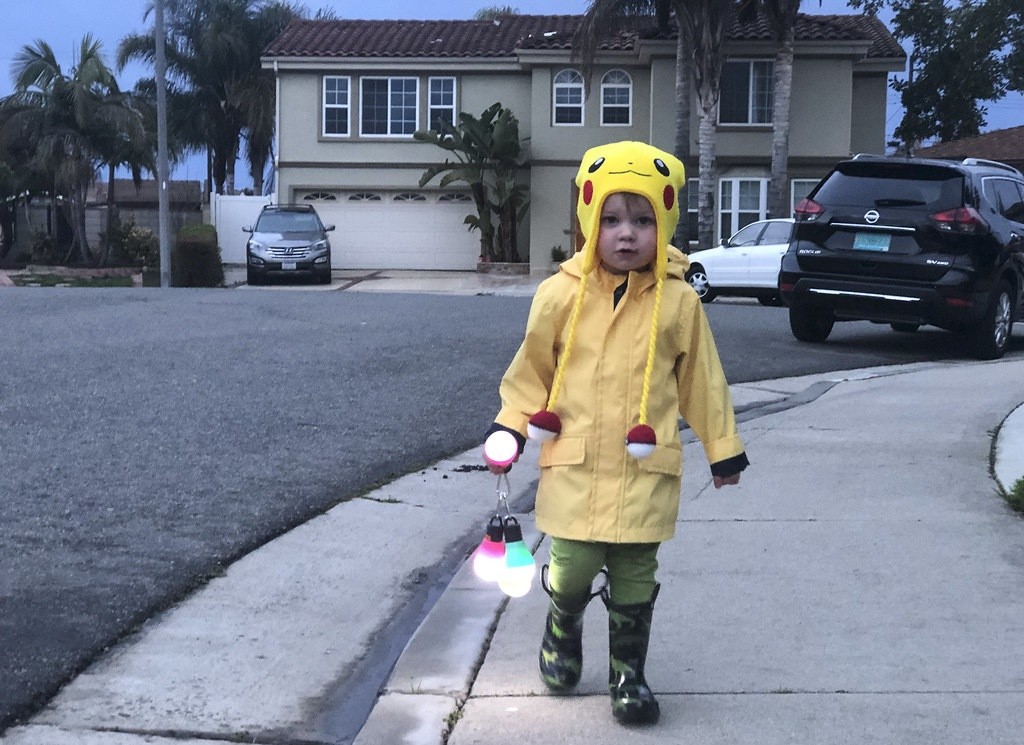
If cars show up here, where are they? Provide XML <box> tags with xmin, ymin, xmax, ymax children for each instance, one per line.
<box><xmin>685</xmin><ymin>218</ymin><xmax>817</xmax><ymax>306</ymax></box>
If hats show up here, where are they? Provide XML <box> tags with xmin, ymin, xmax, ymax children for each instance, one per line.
<box><xmin>576</xmin><ymin>141</ymin><xmax>685</xmax><ymax>281</ymax></box>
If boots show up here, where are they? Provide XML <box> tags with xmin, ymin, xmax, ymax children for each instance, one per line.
<box><xmin>607</xmin><ymin>603</ymin><xmax>661</xmax><ymax>724</ymax></box>
<box><xmin>538</xmin><ymin>586</ymin><xmax>590</xmax><ymax>690</ymax></box>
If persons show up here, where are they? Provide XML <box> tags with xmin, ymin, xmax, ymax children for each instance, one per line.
<box><xmin>479</xmin><ymin>138</ymin><xmax>752</xmax><ymax>725</ymax></box>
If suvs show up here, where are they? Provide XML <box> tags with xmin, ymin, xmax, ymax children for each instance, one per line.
<box><xmin>777</xmin><ymin>153</ymin><xmax>1024</xmax><ymax>360</ymax></box>
<box><xmin>242</xmin><ymin>204</ymin><xmax>336</xmax><ymax>285</ymax></box>
